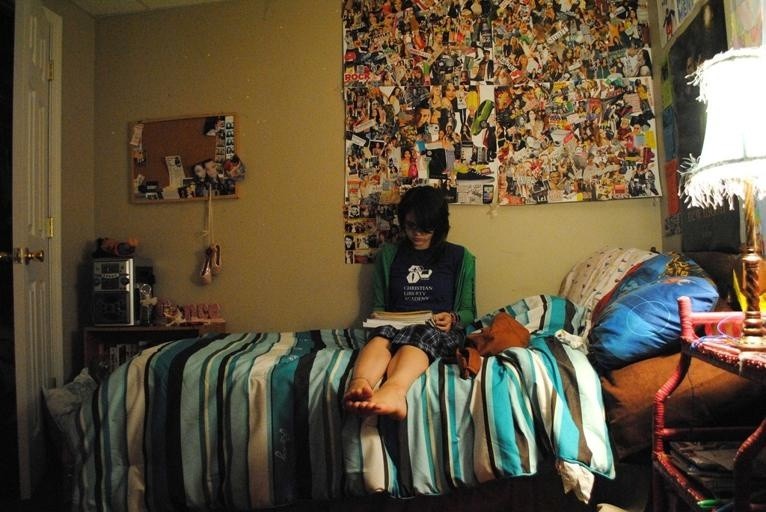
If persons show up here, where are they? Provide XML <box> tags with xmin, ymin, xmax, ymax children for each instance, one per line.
<box><xmin>340</xmin><ymin>188</ymin><xmax>476</xmax><ymax>423</ymax></box>
<box><xmin>342</xmin><ymin>0</ymin><xmax>669</xmax><ymax>265</ymax></box>
<box><xmin>663</xmin><ymin>7</ymin><xmax>676</xmax><ymax>40</ymax></box>
<box><xmin>179</xmin><ymin>116</ymin><xmax>236</xmax><ymax>197</ymax></box>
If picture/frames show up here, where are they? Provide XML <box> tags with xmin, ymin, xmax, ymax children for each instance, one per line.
<box><xmin>128</xmin><ymin>113</ymin><xmax>240</xmax><ymax>205</ymax></box>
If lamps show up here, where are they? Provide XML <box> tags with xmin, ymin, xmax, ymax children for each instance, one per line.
<box><xmin>678</xmin><ymin>45</ymin><xmax>766</xmax><ymax>350</ymax></box>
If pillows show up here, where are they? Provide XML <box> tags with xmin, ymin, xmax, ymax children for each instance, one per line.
<box><xmin>43</xmin><ymin>367</ymin><xmax>96</xmax><ymax>428</ymax></box>
<box><xmin>559</xmin><ymin>246</ymin><xmax>659</xmax><ymax>318</ymax></box>
<box><xmin>586</xmin><ymin>249</ymin><xmax>720</xmax><ymax>369</ymax></box>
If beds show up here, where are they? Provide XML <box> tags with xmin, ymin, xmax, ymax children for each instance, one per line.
<box><xmin>43</xmin><ymin>249</ymin><xmax>766</xmax><ymax>512</ymax></box>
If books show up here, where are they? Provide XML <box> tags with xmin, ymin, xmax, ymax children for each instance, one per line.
<box><xmin>362</xmin><ymin>309</ymin><xmax>436</xmax><ymax>329</ymax></box>
<box><xmin>668</xmin><ymin>437</ymin><xmax>766</xmax><ymax>498</ymax></box>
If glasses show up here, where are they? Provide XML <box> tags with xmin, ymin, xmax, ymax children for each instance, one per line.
<box><xmin>404</xmin><ymin>221</ymin><xmax>439</xmax><ymax>231</ymax></box>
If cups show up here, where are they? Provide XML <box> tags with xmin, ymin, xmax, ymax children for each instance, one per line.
<box><xmin>140</xmin><ymin>284</ymin><xmax>152</xmax><ymax>329</ymax></box>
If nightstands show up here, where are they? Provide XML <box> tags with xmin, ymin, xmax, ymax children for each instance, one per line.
<box><xmin>653</xmin><ymin>297</ymin><xmax>766</xmax><ymax>512</ymax></box>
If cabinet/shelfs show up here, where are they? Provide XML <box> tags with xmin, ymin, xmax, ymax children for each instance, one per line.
<box><xmin>84</xmin><ymin>320</ymin><xmax>226</xmax><ymax>383</ymax></box>
